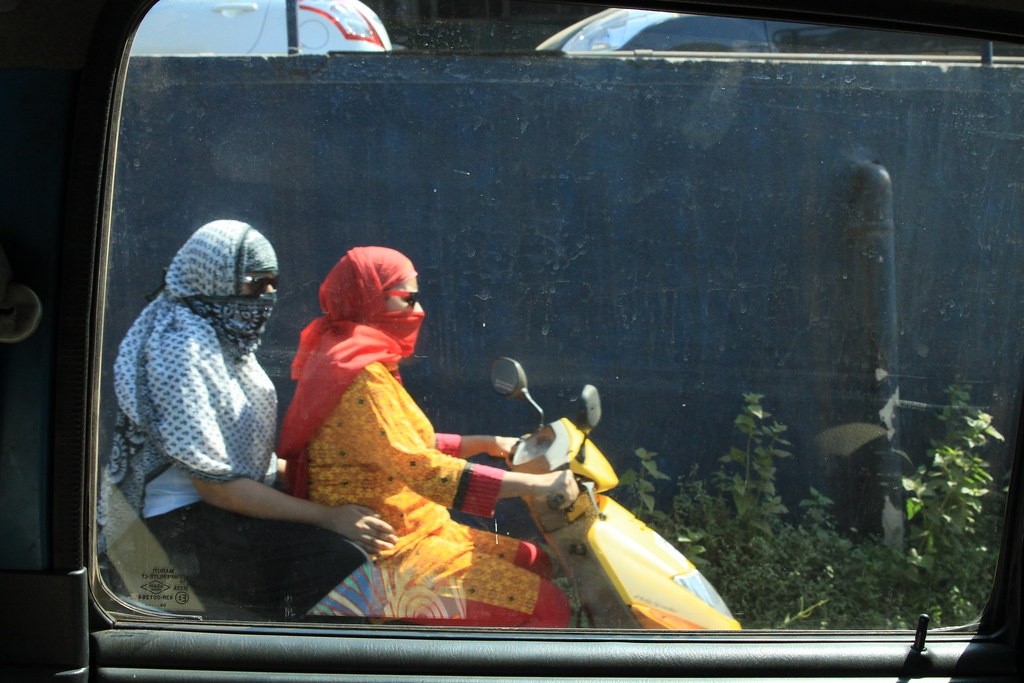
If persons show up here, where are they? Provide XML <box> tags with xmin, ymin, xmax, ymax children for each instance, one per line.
<box><xmin>278</xmin><ymin>246</ymin><xmax>580</xmax><ymax>629</ymax></box>
<box><xmin>103</xmin><ymin>219</ymin><xmax>398</xmax><ymax>624</ymax></box>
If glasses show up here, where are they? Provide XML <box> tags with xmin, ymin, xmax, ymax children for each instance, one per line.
<box><xmin>381</xmin><ymin>291</ymin><xmax>420</xmax><ymax>308</ymax></box>
<box><xmin>242</xmin><ymin>276</ymin><xmax>278</xmax><ymax>295</ymax></box>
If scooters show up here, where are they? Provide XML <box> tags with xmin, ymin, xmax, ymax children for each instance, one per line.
<box><xmin>97</xmin><ymin>355</ymin><xmax>744</xmax><ymax>631</ymax></box>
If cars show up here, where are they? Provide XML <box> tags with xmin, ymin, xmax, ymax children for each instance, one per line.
<box><xmin>529</xmin><ymin>4</ymin><xmax>1024</xmax><ymax>58</ymax></box>
<box><xmin>125</xmin><ymin>0</ymin><xmax>394</xmax><ymax>54</ymax></box>
